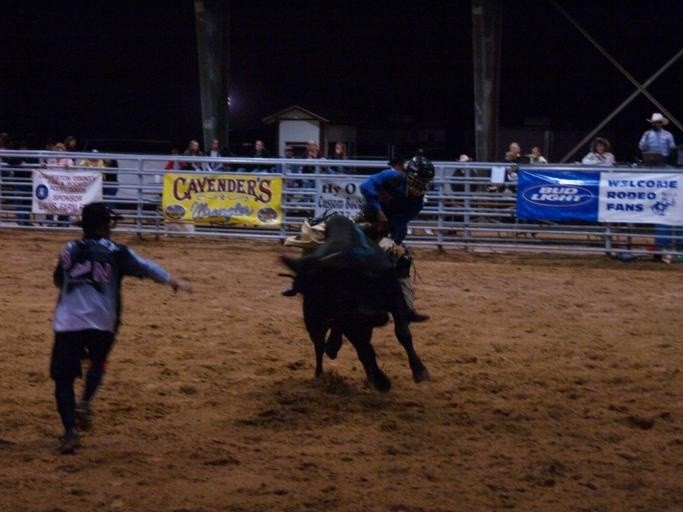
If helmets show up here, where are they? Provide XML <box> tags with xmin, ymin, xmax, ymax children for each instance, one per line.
<box><xmin>405</xmin><ymin>155</ymin><xmax>435</xmax><ymax>190</ymax></box>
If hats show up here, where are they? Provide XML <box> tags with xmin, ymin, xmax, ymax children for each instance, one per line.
<box><xmin>646</xmin><ymin>113</ymin><xmax>668</xmax><ymax>125</ymax></box>
<box><xmin>71</xmin><ymin>202</ymin><xmax>124</xmax><ymax>225</ymax></box>
<box><xmin>590</xmin><ymin>136</ymin><xmax>609</xmax><ymax>153</ymax></box>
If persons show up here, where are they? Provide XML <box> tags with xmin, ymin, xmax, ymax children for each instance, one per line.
<box><xmin>284</xmin><ymin>140</ymin><xmax>357</xmax><ymax>202</ymax></box>
<box><xmin>503</xmin><ymin>142</ymin><xmax>547</xmax><ymax>192</ymax></box>
<box><xmin>449</xmin><ymin>154</ymin><xmax>478</xmax><ymax>192</ymax></box>
<box><xmin>166</xmin><ymin>139</ymin><xmax>224</xmax><ymax>172</ymax></box>
<box><xmin>640</xmin><ymin>112</ymin><xmax>675</xmax><ymax>165</ymax></box>
<box><xmin>278</xmin><ymin>154</ymin><xmax>435</xmax><ymax>324</ymax></box>
<box><xmin>46</xmin><ymin>201</ymin><xmax>195</xmax><ymax>453</ymax></box>
<box><xmin>248</xmin><ymin>140</ymin><xmax>272</xmax><ymax>173</ymax></box>
<box><xmin>582</xmin><ymin>138</ymin><xmax>615</xmax><ymax>166</ymax></box>
<box><xmin>41</xmin><ymin>136</ymin><xmax>105</xmax><ymax>167</ymax></box>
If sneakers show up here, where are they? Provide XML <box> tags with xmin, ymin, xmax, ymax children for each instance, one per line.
<box><xmin>56</xmin><ymin>403</ymin><xmax>94</xmax><ymax>453</ymax></box>
<box><xmin>409</xmin><ymin>313</ymin><xmax>429</xmax><ymax>324</ymax></box>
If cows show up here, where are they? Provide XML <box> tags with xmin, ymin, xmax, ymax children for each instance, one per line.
<box><xmin>281</xmin><ymin>212</ymin><xmax>434</xmax><ymax>393</ymax></box>
